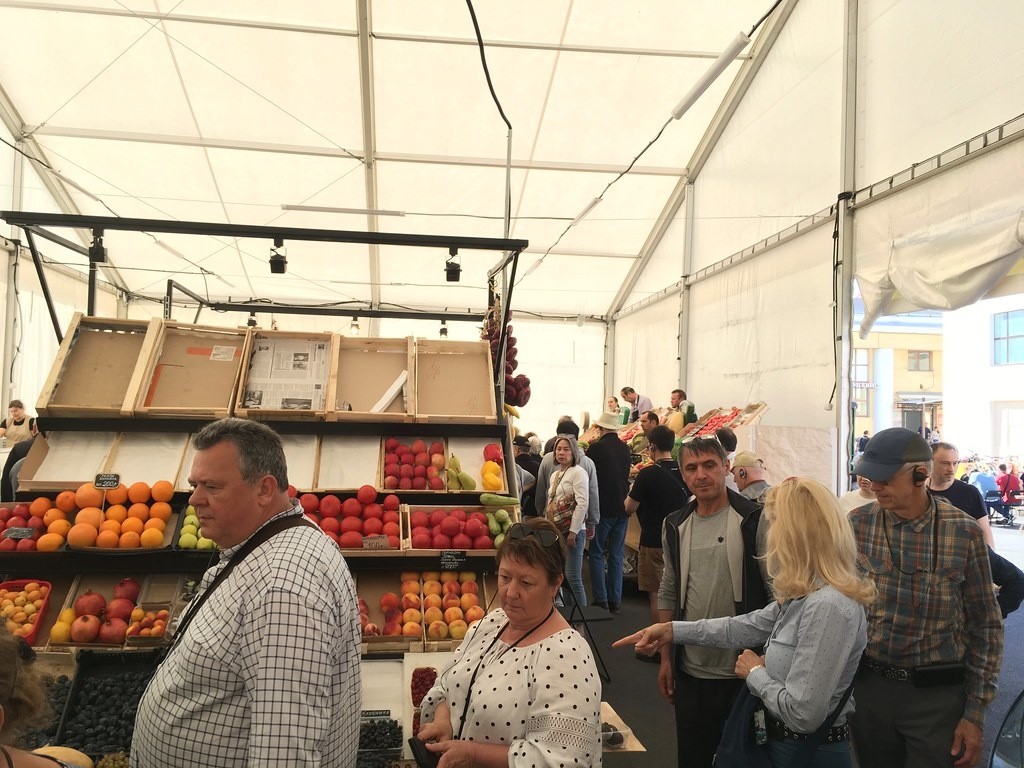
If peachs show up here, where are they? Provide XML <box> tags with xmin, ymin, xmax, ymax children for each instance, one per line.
<box><xmin>126</xmin><ymin>608</ymin><xmax>169</xmax><ymax>636</ymax></box>
<box><xmin>358</xmin><ymin>571</ymin><xmax>485</xmax><ymax>638</ymax></box>
<box><xmin>383</xmin><ymin>439</ymin><xmax>446</xmax><ymax>490</ymax></box>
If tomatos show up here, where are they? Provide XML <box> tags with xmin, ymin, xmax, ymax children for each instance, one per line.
<box><xmin>285</xmin><ymin>484</ymin><xmax>493</xmax><ymax>550</ymax></box>
<box><xmin>620</xmin><ymin>409</ymin><xmax>748</xmax><ymax>442</ymax></box>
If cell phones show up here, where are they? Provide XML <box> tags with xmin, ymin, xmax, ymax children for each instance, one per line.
<box><xmin>754</xmin><ymin>709</ymin><xmax>767</xmax><ymax>746</ymax></box>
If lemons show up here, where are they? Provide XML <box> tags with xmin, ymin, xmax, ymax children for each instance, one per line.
<box><xmin>51</xmin><ymin>608</ymin><xmax>76</xmax><ymax>643</ymax></box>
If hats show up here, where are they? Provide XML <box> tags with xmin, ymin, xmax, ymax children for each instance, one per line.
<box><xmin>730</xmin><ymin>450</ymin><xmax>764</xmax><ymax>471</ymax></box>
<box><xmin>513</xmin><ymin>436</ymin><xmax>531</xmax><ymax>447</ymax></box>
<box><xmin>849</xmin><ymin>427</ymin><xmax>933</xmax><ymax>482</ymax></box>
<box><xmin>595</xmin><ymin>412</ymin><xmax>619</xmax><ymax>430</ymax></box>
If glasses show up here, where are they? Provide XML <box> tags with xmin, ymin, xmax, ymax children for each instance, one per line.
<box><xmin>646</xmin><ymin>443</ymin><xmax>654</xmax><ymax>451</ymax></box>
<box><xmin>681</xmin><ymin>434</ymin><xmax>722</xmax><ymax>447</ymax></box>
<box><xmin>505</xmin><ymin>523</ymin><xmax>563</xmax><ymax>564</ymax></box>
<box><xmin>557</xmin><ymin>433</ymin><xmax>576</xmax><ymax>440</ymax></box>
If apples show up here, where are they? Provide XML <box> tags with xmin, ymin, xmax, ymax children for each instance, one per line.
<box><xmin>179</xmin><ymin>504</ymin><xmax>223</xmax><ymax>549</ymax></box>
<box><xmin>0</xmin><ymin>503</ymin><xmax>45</xmax><ymax>552</ymax></box>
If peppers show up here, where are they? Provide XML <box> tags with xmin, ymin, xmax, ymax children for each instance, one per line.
<box><xmin>481</xmin><ymin>443</ymin><xmax>503</xmax><ymax>490</ymax></box>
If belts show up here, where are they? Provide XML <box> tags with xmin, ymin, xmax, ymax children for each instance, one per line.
<box><xmin>866</xmin><ymin>660</ymin><xmax>915</xmax><ymax>681</ymax></box>
<box><xmin>771</xmin><ymin>720</ymin><xmax>849</xmax><ymax>743</ymax></box>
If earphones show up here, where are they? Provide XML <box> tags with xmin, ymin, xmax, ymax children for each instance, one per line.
<box><xmin>740</xmin><ymin>470</ymin><xmax>744</xmax><ymax>476</ymax></box>
<box><xmin>649</xmin><ymin>445</ymin><xmax>654</xmax><ymax>451</ymax></box>
<box><xmin>913</xmin><ymin>471</ymin><xmax>926</xmax><ymax>483</ymax></box>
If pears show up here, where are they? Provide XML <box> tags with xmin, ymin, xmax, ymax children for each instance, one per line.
<box><xmin>445</xmin><ymin>454</ymin><xmax>476</xmax><ymax>490</ymax></box>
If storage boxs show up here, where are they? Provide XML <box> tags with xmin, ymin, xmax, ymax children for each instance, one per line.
<box><xmin>358</xmin><ymin>747</ymin><xmax>403</xmax><ymax>761</ymax></box>
<box><xmin>53</xmin><ymin>654</ymin><xmax>163</xmax><ymax>754</ymax></box>
<box><xmin>603</xmin><ymin>718</ymin><xmax>631</xmax><ymax>750</ymax></box>
<box><xmin>0</xmin><ymin>312</ymin><xmax>522</xmax><ymax>662</ymax></box>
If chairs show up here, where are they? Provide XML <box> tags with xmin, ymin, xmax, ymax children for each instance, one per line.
<box><xmin>984</xmin><ymin>490</ymin><xmax>1024</xmax><ymax>527</ymax></box>
<box><xmin>554</xmin><ymin>575</ymin><xmax>615</xmax><ymax>683</ymax></box>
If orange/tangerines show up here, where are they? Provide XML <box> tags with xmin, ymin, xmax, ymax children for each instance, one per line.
<box><xmin>30</xmin><ymin>482</ymin><xmax>174</xmax><ymax>550</ymax></box>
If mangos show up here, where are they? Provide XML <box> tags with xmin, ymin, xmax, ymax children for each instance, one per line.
<box><xmin>0</xmin><ymin>582</ymin><xmax>47</xmax><ymax>636</ymax></box>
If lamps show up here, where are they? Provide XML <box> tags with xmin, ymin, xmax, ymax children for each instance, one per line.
<box><xmin>269</xmin><ymin>237</ymin><xmax>288</xmax><ymax>273</ymax></box>
<box><xmin>444</xmin><ymin>247</ymin><xmax>463</xmax><ymax>282</ymax></box>
<box><xmin>90</xmin><ymin>228</ymin><xmax>108</xmax><ymax>263</ymax></box>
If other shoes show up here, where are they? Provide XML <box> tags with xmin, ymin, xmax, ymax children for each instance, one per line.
<box><xmin>591</xmin><ymin>602</ymin><xmax>608</xmax><ymax>611</ymax></box>
<box><xmin>1006</xmin><ymin>515</ymin><xmax>1016</xmax><ymax>525</ymax></box>
<box><xmin>608</xmin><ymin>601</ymin><xmax>620</xmax><ymax>613</ymax></box>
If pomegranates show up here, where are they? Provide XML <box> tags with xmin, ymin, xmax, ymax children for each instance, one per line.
<box><xmin>71</xmin><ymin>577</ymin><xmax>141</xmax><ymax>644</ymax></box>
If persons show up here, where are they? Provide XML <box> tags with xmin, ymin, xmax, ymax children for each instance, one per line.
<box><xmin>415</xmin><ymin>517</ymin><xmax>602</xmax><ymax>767</ymax></box>
<box><xmin>513</xmin><ymin>387</ymin><xmax>773</xmax><ymax>634</ymax></box>
<box><xmin>129</xmin><ymin>417</ymin><xmax>363</xmax><ymax>768</ymax></box>
<box><xmin>0</xmin><ymin>619</ymin><xmax>69</xmax><ymax>768</ymax></box>
<box><xmin>612</xmin><ymin>435</ymin><xmax>878</xmax><ymax>768</ymax></box>
<box><xmin>846</xmin><ymin>428</ymin><xmax>1006</xmax><ymax>768</ymax></box>
<box><xmin>0</xmin><ymin>418</ymin><xmax>55</xmax><ymax>503</ymax></box>
<box><xmin>926</xmin><ymin>442</ymin><xmax>1024</xmax><ymax>620</ymax></box>
<box><xmin>543</xmin><ymin>433</ymin><xmax>591</xmax><ymax>638</ymax></box>
<box><xmin>851</xmin><ymin>430</ymin><xmax>1024</xmax><ymax>524</ymax></box>
<box><xmin>0</xmin><ymin>400</ymin><xmax>35</xmax><ymax>444</ymax></box>
